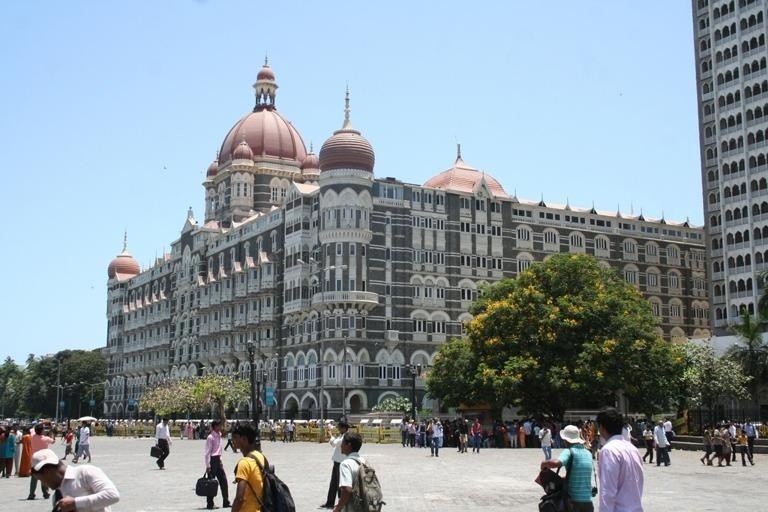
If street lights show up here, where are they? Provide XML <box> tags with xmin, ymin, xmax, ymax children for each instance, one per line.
<box><xmin>406</xmin><ymin>362</ymin><xmax>422</xmax><ymax>422</ymax></box>
<box><xmin>245</xmin><ymin>339</ymin><xmax>256</xmax><ymax>426</ymax></box>
<box><xmin>80</xmin><ymin>379</ymin><xmax>109</xmax><ymax>418</ymax></box>
<box><xmin>51</xmin><ymin>381</ymin><xmax>84</xmax><ymax>421</ymax></box>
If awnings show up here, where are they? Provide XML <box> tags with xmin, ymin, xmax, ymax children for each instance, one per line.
<box><xmin>359</xmin><ymin>419</ymin><xmax>402</xmax><ymax>424</ymax></box>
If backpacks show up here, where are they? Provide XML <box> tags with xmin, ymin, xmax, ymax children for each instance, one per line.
<box><xmin>349</xmin><ymin>457</ymin><xmax>383</xmax><ymax>512</ymax></box>
<box><xmin>234</xmin><ymin>453</ymin><xmax>295</xmax><ymax>512</ymax></box>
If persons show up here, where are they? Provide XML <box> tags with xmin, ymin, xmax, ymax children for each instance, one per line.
<box><xmin>540</xmin><ymin>425</ymin><xmax>594</xmax><ymax>511</ymax></box>
<box><xmin>89</xmin><ymin>420</ymin><xmax>95</xmax><ymax>436</ymax></box>
<box><xmin>490</xmin><ymin>414</ymin><xmax>673</xmax><ymax>466</ymax></box>
<box><xmin>320</xmin><ymin>422</ymin><xmax>367</xmax><ymax>511</ymax></box>
<box><xmin>700</xmin><ymin>417</ymin><xmax>768</xmax><ymax>467</ymax></box>
<box><xmin>596</xmin><ymin>414</ymin><xmax>644</xmax><ymax>511</ymax></box>
<box><xmin>180</xmin><ymin>418</ymin><xmax>296</xmax><ymax>453</ymax></box>
<box><xmin>31</xmin><ymin>449</ymin><xmax>120</xmax><ymax>511</ymax></box>
<box><xmin>205</xmin><ymin>419</ymin><xmax>232</xmax><ymax>510</ymax></box>
<box><xmin>156</xmin><ymin>416</ymin><xmax>173</xmax><ymax>470</ymax></box>
<box><xmin>0</xmin><ymin>418</ymin><xmax>92</xmax><ymax>500</ymax></box>
<box><xmin>302</xmin><ymin>420</ymin><xmax>334</xmax><ymax>428</ymax></box>
<box><xmin>106</xmin><ymin>419</ymin><xmax>113</xmax><ymax>436</ymax></box>
<box><xmin>231</xmin><ymin>426</ymin><xmax>264</xmax><ymax>511</ymax></box>
<box><xmin>399</xmin><ymin>417</ymin><xmax>491</xmax><ymax>457</ymax></box>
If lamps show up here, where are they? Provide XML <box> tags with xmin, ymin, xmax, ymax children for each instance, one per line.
<box><xmin>296</xmin><ymin>258</ymin><xmax>308</xmax><ymax>267</ymax></box>
<box><xmin>308</xmin><ymin>255</ymin><xmax>319</xmax><ymax>266</ymax></box>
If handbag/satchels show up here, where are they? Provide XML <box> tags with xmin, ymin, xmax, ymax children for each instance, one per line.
<box><xmin>195</xmin><ymin>478</ymin><xmax>218</xmax><ymax>497</ymax></box>
<box><xmin>150</xmin><ymin>447</ymin><xmax>163</xmax><ymax>457</ymax></box>
<box><xmin>539</xmin><ymin>491</ymin><xmax>573</xmax><ymax>511</ymax></box>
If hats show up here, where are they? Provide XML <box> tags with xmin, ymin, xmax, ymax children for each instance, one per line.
<box><xmin>31</xmin><ymin>449</ymin><xmax>59</xmax><ymax>471</ymax></box>
<box><xmin>560</xmin><ymin>425</ymin><xmax>586</xmax><ymax>444</ymax></box>
